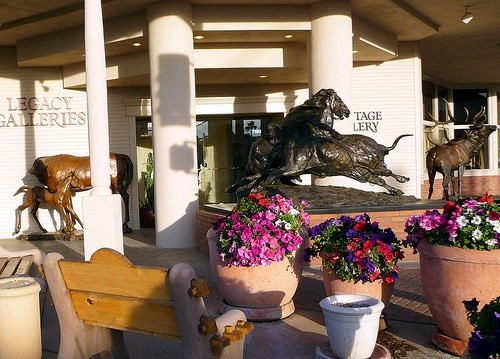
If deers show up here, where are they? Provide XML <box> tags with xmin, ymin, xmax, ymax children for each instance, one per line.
<box><xmin>423</xmin><ymin>98</ymin><xmax>498</xmax><ymax>201</ymax></box>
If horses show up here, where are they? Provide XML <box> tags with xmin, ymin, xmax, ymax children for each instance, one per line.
<box><xmin>13</xmin><ymin>169</ymin><xmax>84</xmax><ymax>235</ymax></box>
<box><xmin>29</xmin><ymin>153</ymin><xmax>134</xmax><ymax>235</ymax></box>
<box><xmin>227</xmin><ymin>87</ymin><xmax>409</xmax><ymax>196</ymax></box>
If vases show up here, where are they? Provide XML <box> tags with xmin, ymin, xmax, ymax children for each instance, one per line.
<box><xmin>319</xmin><ymin>294</ymin><xmax>386</xmax><ymax>359</ymax></box>
<box><xmin>322</xmin><ymin>258</ymin><xmax>395</xmax><ymax>311</ymax></box>
<box><xmin>418</xmin><ymin>240</ymin><xmax>500</xmax><ymax>342</ymax></box>
<box><xmin>0</xmin><ymin>277</ymin><xmax>47</xmax><ymax>359</ymax></box>
<box><xmin>206</xmin><ymin>228</ymin><xmax>309</xmax><ymax>308</ymax></box>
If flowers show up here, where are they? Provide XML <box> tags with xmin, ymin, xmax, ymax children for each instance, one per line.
<box><xmin>403</xmin><ymin>190</ymin><xmax>500</xmax><ymax>254</ymax></box>
<box><xmin>212</xmin><ymin>188</ymin><xmax>309</xmax><ymax>268</ymax></box>
<box><xmin>462</xmin><ymin>297</ymin><xmax>500</xmax><ymax>359</ymax></box>
<box><xmin>304</xmin><ymin>213</ymin><xmax>405</xmax><ymax>284</ymax></box>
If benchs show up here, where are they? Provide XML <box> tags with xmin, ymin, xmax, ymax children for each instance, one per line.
<box><xmin>0</xmin><ymin>255</ymin><xmax>34</xmax><ymax>279</ymax></box>
<box><xmin>42</xmin><ymin>248</ymin><xmax>253</xmax><ymax>359</ymax></box>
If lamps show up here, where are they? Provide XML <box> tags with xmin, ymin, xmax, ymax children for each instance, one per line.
<box><xmin>461</xmin><ymin>4</ymin><xmax>474</xmax><ymax>24</ymax></box>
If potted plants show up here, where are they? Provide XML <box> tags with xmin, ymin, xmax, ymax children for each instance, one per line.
<box><xmin>139</xmin><ymin>152</ymin><xmax>155</xmax><ymax>228</ymax></box>
